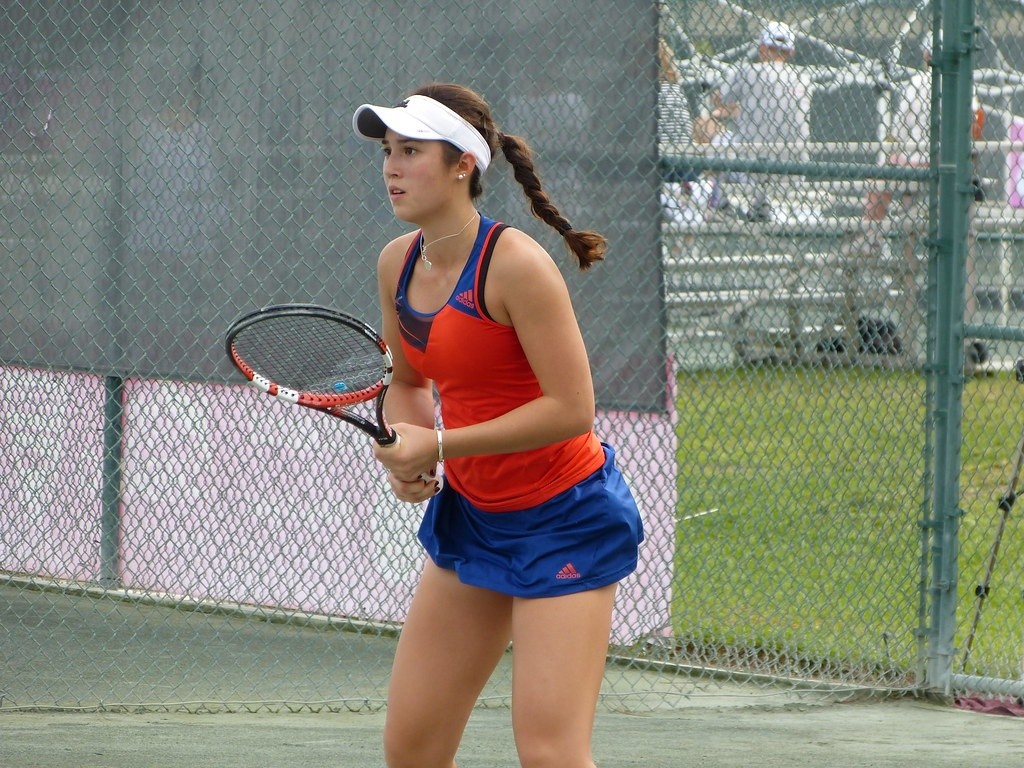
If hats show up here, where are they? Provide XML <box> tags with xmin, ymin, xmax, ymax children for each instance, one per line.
<box><xmin>919</xmin><ymin>27</ymin><xmax>944</xmax><ymax>57</ymax></box>
<box><xmin>352</xmin><ymin>94</ymin><xmax>492</xmax><ymax>177</ymax></box>
<box><xmin>759</xmin><ymin>20</ymin><xmax>796</xmax><ymax>50</ymax></box>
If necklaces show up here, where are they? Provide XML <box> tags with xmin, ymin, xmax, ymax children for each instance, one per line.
<box><xmin>420</xmin><ymin>210</ymin><xmax>478</xmax><ymax>270</ymax></box>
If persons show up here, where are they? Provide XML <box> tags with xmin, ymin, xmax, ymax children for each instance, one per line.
<box><xmin>352</xmin><ymin>82</ymin><xmax>645</xmax><ymax>768</ymax></box>
<box><xmin>516</xmin><ymin>24</ymin><xmax>987</xmax><ymax>252</ymax></box>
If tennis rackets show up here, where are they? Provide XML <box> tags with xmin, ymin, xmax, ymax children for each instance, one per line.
<box><xmin>224</xmin><ymin>303</ymin><xmax>400</xmax><ymax>449</ymax></box>
